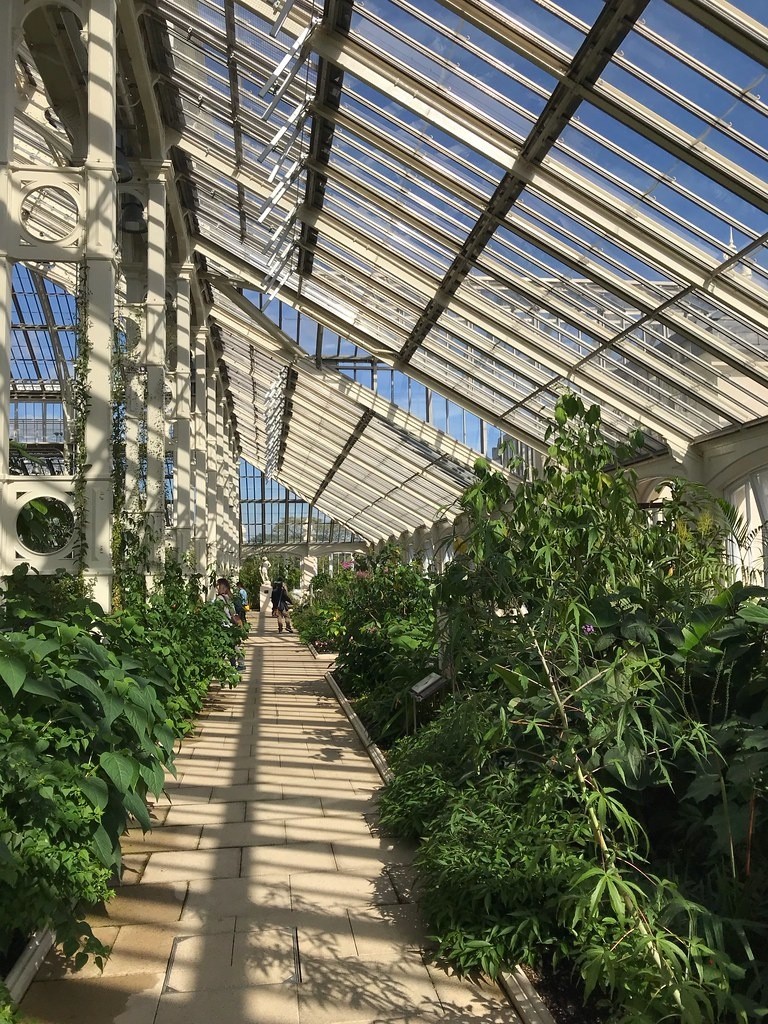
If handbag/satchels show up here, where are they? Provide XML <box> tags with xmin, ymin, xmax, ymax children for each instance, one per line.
<box><xmin>231</xmin><ymin>614</ymin><xmax>242</xmax><ymax>629</ymax></box>
<box><xmin>272</xmin><ymin>609</ymin><xmax>279</xmax><ymax>618</ymax></box>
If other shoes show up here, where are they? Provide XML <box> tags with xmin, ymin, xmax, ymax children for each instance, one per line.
<box><xmin>237</xmin><ymin>666</ymin><xmax>246</xmax><ymax>671</ymax></box>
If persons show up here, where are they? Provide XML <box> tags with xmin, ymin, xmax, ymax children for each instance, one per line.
<box><xmin>214</xmin><ymin>578</ymin><xmax>248</xmax><ymax>631</ymax></box>
<box><xmin>271</xmin><ymin>576</ymin><xmax>295</xmax><ymax>633</ymax></box>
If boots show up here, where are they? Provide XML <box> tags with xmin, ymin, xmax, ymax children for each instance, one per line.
<box><xmin>286</xmin><ymin>622</ymin><xmax>293</xmax><ymax>633</ymax></box>
<box><xmin>279</xmin><ymin>623</ymin><xmax>282</xmax><ymax>633</ymax></box>
